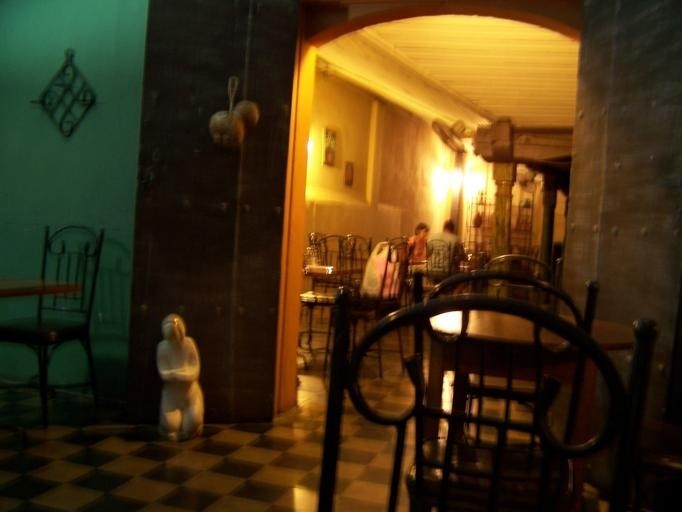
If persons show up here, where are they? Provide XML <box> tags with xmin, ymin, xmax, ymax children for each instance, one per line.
<box><xmin>426</xmin><ymin>218</ymin><xmax>466</xmax><ymax>280</ymax></box>
<box><xmin>406</xmin><ymin>222</ymin><xmax>429</xmax><ymax>262</ymax></box>
<box><xmin>154</xmin><ymin>313</ymin><xmax>206</xmax><ymax>441</ymax></box>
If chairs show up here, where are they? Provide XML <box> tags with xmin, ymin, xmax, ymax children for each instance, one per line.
<box><xmin>0</xmin><ymin>221</ymin><xmax>108</xmax><ymax>430</ymax></box>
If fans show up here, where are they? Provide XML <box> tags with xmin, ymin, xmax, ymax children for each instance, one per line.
<box><xmin>431</xmin><ymin>118</ymin><xmax>476</xmax><ymax>155</ymax></box>
